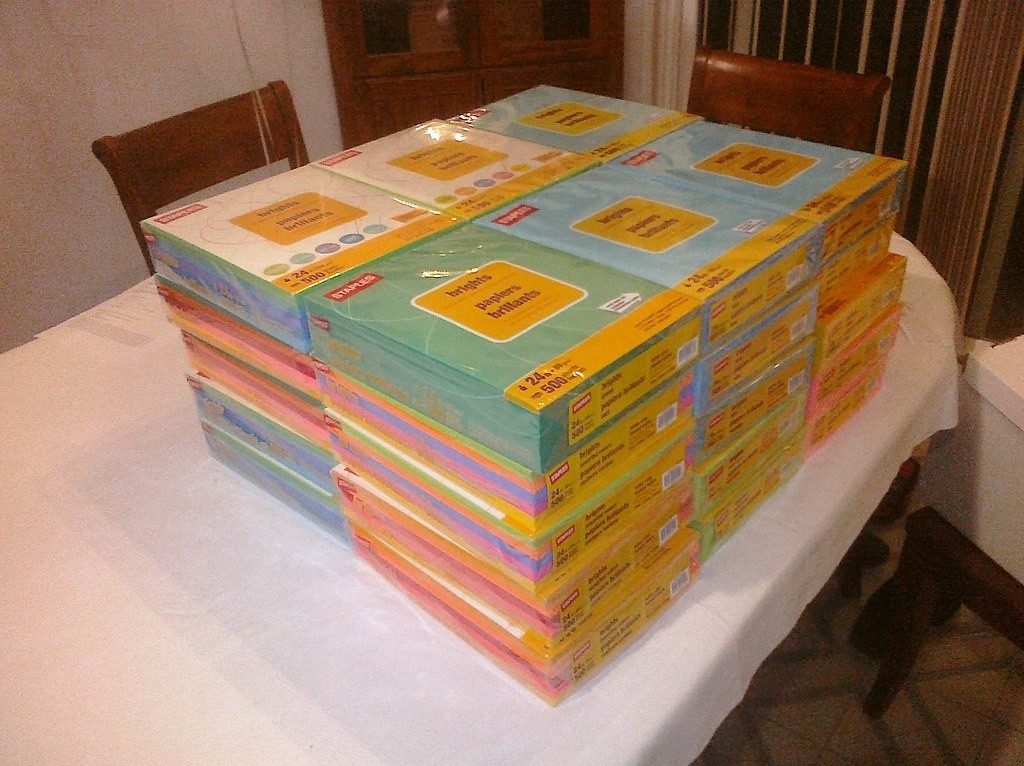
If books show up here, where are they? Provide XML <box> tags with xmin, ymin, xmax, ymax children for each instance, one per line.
<box><xmin>137</xmin><ymin>84</ymin><xmax>914</xmax><ymax>708</ymax></box>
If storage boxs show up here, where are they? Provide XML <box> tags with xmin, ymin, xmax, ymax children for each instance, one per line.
<box><xmin>920</xmin><ymin>334</ymin><xmax>1024</xmax><ymax>586</ymax></box>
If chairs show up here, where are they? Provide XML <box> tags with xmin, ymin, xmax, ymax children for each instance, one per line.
<box><xmin>90</xmin><ymin>81</ymin><xmax>312</xmax><ymax>276</ymax></box>
<box><xmin>863</xmin><ymin>506</ymin><xmax>1024</xmax><ymax>721</ymax></box>
<box><xmin>686</xmin><ymin>45</ymin><xmax>892</xmax><ymax>152</ymax></box>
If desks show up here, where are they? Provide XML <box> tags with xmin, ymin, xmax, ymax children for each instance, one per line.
<box><xmin>0</xmin><ymin>229</ymin><xmax>960</xmax><ymax>766</ymax></box>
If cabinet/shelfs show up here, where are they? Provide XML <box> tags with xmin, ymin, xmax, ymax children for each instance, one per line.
<box><xmin>321</xmin><ymin>0</ymin><xmax>624</xmax><ymax>146</ymax></box>
